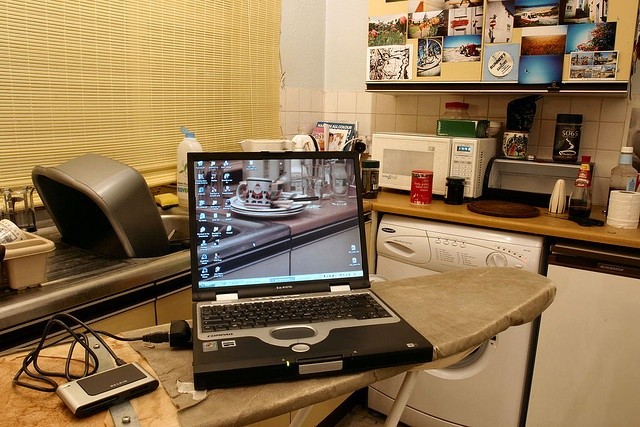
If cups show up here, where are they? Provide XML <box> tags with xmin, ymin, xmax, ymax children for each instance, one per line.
<box><xmin>302</xmin><ymin>163</ymin><xmax>323</xmax><ymax>179</ymax></box>
<box><xmin>409</xmin><ymin>168</ymin><xmax>434</xmax><ymax>207</ymax></box>
<box><xmin>503</xmin><ymin>131</ymin><xmax>529</xmax><ymax>161</ymax></box>
<box><xmin>236</xmin><ymin>177</ymin><xmax>272</xmax><ymax>208</ymax></box>
<box><xmin>303</xmin><ymin>177</ymin><xmax>323</xmax><ymax>207</ymax></box>
<box><xmin>330</xmin><ymin>163</ymin><xmax>350</xmax><ymax>206</ymax></box>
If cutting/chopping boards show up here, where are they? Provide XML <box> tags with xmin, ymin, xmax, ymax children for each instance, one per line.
<box><xmin>466</xmin><ymin>200</ymin><xmax>541</xmax><ymax>218</ymax></box>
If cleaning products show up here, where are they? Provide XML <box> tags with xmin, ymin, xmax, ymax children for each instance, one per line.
<box><xmin>176</xmin><ymin>127</ymin><xmax>206</xmax><ymax>213</ymax></box>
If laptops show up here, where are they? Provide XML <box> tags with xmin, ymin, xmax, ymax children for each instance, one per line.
<box><xmin>188</xmin><ymin>151</ymin><xmax>432</xmax><ymax>390</ymax></box>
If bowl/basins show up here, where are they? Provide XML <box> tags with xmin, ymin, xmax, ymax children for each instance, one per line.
<box><xmin>271</xmin><ymin>181</ymin><xmax>285</xmax><ymax>201</ymax></box>
<box><xmin>487</xmin><ymin>120</ymin><xmax>503</xmax><ymax>139</ymax></box>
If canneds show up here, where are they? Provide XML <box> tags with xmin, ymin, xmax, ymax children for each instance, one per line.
<box><xmin>359</xmin><ymin>160</ymin><xmax>381</xmax><ymax>198</ymax></box>
<box><xmin>444</xmin><ymin>175</ymin><xmax>465</xmax><ymax>203</ymax></box>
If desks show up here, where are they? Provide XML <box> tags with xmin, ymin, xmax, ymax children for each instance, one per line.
<box><xmin>0</xmin><ymin>267</ymin><xmax>557</xmax><ymax>427</ymax></box>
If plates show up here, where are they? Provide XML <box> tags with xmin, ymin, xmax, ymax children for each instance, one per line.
<box><xmin>228</xmin><ymin>209</ymin><xmax>303</xmax><ymax>219</ymax></box>
<box><xmin>230</xmin><ymin>206</ymin><xmax>305</xmax><ymax>213</ymax></box>
<box><xmin>230</xmin><ymin>196</ymin><xmax>307</xmax><ymax>209</ymax></box>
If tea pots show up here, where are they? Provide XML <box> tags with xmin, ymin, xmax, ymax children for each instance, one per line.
<box><xmin>238</xmin><ymin>139</ymin><xmax>294</xmax><ymax>152</ymax></box>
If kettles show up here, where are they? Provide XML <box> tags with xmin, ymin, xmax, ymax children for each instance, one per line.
<box><xmin>291</xmin><ymin>159</ymin><xmax>322</xmax><ymax>187</ymax></box>
<box><xmin>293</xmin><ymin>134</ymin><xmax>320</xmax><ymax>151</ymax></box>
<box><xmin>237</xmin><ymin>160</ymin><xmax>294</xmax><ymax>194</ymax></box>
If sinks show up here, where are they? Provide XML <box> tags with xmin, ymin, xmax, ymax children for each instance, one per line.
<box><xmin>51</xmin><ymin>213</ymin><xmax>189</xmax><ymax>263</ymax></box>
<box><xmin>197</xmin><ymin>208</ymin><xmax>267</xmax><ymax>258</ymax></box>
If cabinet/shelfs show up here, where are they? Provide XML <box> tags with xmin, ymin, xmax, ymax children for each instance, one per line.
<box><xmin>0</xmin><ymin>273</ymin><xmax>195</xmax><ymax>353</ymax></box>
<box><xmin>202</xmin><ymin>220</ymin><xmax>365</xmax><ymax>280</ymax></box>
<box><xmin>365</xmin><ymin>1</ymin><xmax>638</xmax><ymax>93</ymax></box>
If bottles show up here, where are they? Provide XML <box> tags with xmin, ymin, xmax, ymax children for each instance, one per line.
<box><xmin>605</xmin><ymin>145</ymin><xmax>639</xmax><ymax>221</ymax></box>
<box><xmin>437</xmin><ymin>101</ymin><xmax>472</xmax><ymax>138</ymax></box>
<box><xmin>568</xmin><ymin>154</ymin><xmax>594</xmax><ymax>223</ymax></box>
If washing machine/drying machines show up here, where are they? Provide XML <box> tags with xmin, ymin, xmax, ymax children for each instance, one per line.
<box><xmin>367</xmin><ymin>216</ymin><xmax>542</xmax><ymax>427</ymax></box>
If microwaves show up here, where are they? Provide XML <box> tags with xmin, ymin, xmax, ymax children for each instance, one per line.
<box><xmin>369</xmin><ymin>132</ymin><xmax>502</xmax><ymax>204</ymax></box>
